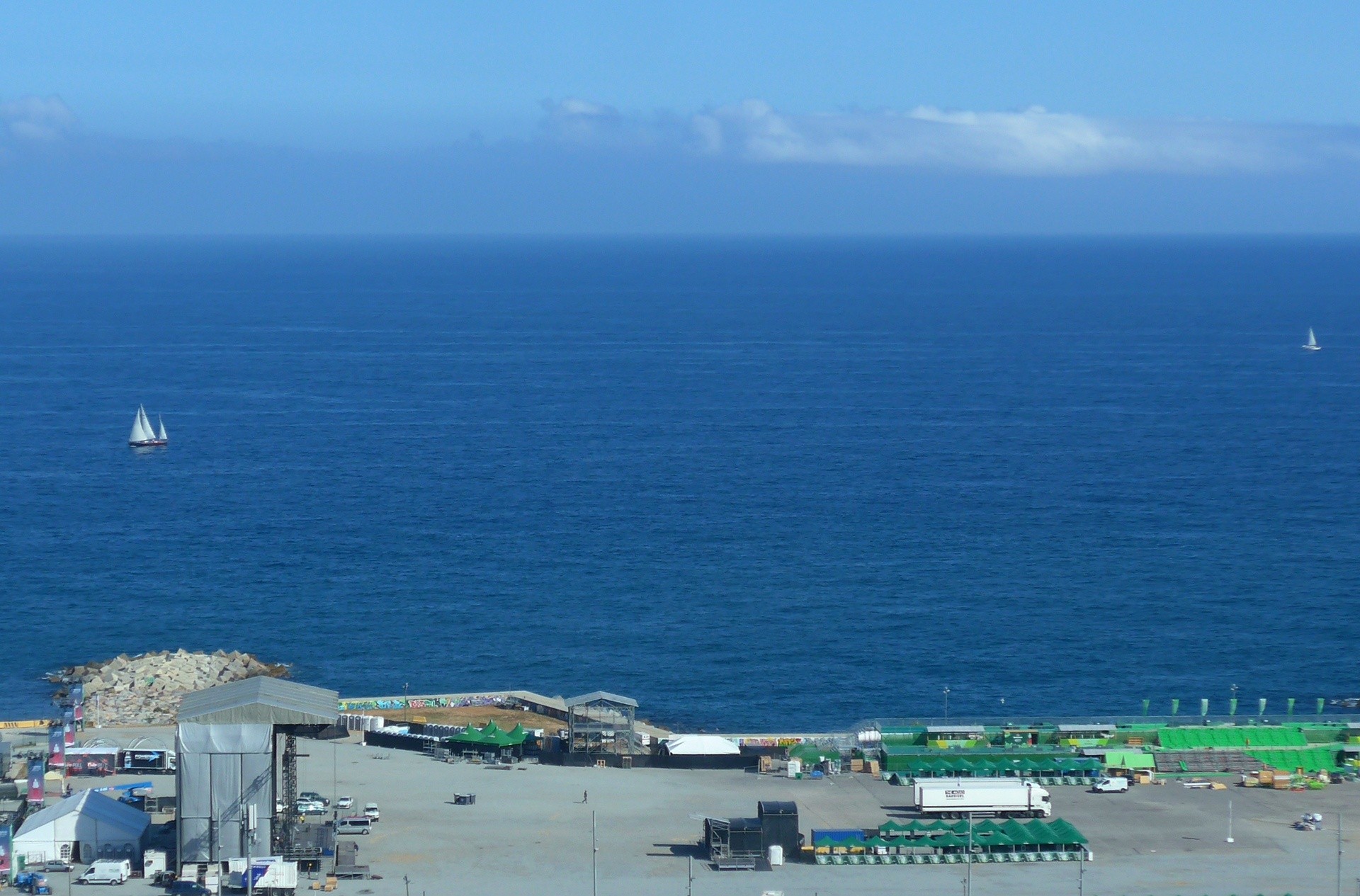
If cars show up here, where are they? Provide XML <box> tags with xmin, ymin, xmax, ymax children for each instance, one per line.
<box><xmin>336</xmin><ymin>796</ymin><xmax>354</xmax><ymax>809</ymax></box>
<box><xmin>1122</xmin><ymin>774</ymin><xmax>1135</xmax><ymax>787</ymax></box>
<box><xmin>154</xmin><ymin>871</ymin><xmax>176</xmax><ymax>887</ymax></box>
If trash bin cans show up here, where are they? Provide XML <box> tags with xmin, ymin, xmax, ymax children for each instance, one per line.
<box><xmin>459</xmin><ymin>795</ymin><xmax>470</xmax><ymax>805</ymax></box>
<box><xmin>795</xmin><ymin>772</ymin><xmax>802</xmax><ymax>780</ymax></box>
<box><xmin>468</xmin><ymin>793</ymin><xmax>476</xmax><ymax>805</ymax></box>
<box><xmin>454</xmin><ymin>793</ymin><xmax>460</xmax><ymax>804</ymax></box>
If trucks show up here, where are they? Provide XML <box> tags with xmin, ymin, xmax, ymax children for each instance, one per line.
<box><xmin>78</xmin><ymin>858</ymin><xmax>131</xmax><ymax>885</ymax></box>
<box><xmin>1092</xmin><ymin>777</ymin><xmax>1128</xmax><ymax>794</ymax></box>
<box><xmin>122</xmin><ymin>748</ymin><xmax>176</xmax><ymax>775</ymax></box>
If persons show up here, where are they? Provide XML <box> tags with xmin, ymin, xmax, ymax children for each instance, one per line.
<box><xmin>582</xmin><ymin>790</ymin><xmax>588</xmax><ymax>804</ymax></box>
<box><xmin>66</xmin><ymin>783</ymin><xmax>71</xmax><ymax>798</ymax></box>
<box><xmin>299</xmin><ymin>816</ymin><xmax>305</xmax><ymax>823</ymax></box>
<box><xmin>354</xmin><ymin>842</ymin><xmax>359</xmax><ymax>857</ymax></box>
<box><xmin>334</xmin><ymin>811</ymin><xmax>337</xmax><ymax>820</ymax></box>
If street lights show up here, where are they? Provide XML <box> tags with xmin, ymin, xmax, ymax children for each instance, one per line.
<box><xmin>402</xmin><ymin>682</ymin><xmax>408</xmax><ymax>722</ymax></box>
<box><xmin>943</xmin><ymin>686</ymin><xmax>951</xmax><ymax>725</ymax></box>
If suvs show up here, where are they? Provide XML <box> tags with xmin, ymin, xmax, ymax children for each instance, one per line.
<box><xmin>43</xmin><ymin>860</ymin><xmax>74</xmax><ymax>873</ymax></box>
<box><xmin>276</xmin><ymin>792</ymin><xmax>331</xmax><ymax>816</ymax></box>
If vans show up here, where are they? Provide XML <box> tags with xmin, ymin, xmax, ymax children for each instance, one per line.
<box><xmin>363</xmin><ymin>803</ymin><xmax>380</xmax><ymax>822</ymax></box>
<box><xmin>335</xmin><ymin>817</ymin><xmax>371</xmax><ymax>835</ymax></box>
<box><xmin>170</xmin><ymin>880</ymin><xmax>212</xmax><ymax>896</ymax></box>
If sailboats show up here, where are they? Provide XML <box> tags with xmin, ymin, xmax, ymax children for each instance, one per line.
<box><xmin>128</xmin><ymin>402</ymin><xmax>169</xmax><ymax>446</ymax></box>
<box><xmin>1301</xmin><ymin>326</ymin><xmax>1323</xmax><ymax>351</ymax></box>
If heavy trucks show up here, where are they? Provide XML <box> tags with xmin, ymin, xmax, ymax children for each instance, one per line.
<box><xmin>912</xmin><ymin>776</ymin><xmax>1052</xmax><ymax>820</ymax></box>
